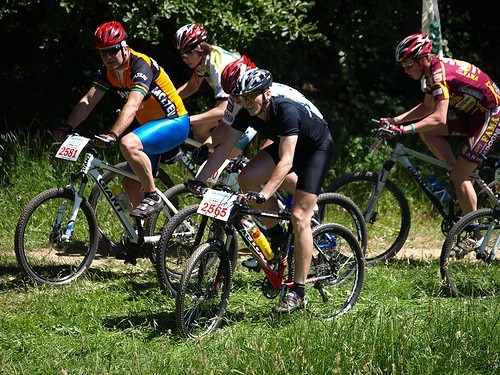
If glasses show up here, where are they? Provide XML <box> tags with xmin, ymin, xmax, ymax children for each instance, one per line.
<box><xmin>401</xmin><ymin>62</ymin><xmax>414</xmax><ymax>68</ymax></box>
<box><xmin>98</xmin><ymin>47</ymin><xmax>122</xmax><ymax>58</ymax></box>
<box><xmin>239</xmin><ymin>91</ymin><xmax>262</xmax><ymax>103</ymax></box>
<box><xmin>179</xmin><ymin>48</ymin><xmax>195</xmax><ymax>58</ymax></box>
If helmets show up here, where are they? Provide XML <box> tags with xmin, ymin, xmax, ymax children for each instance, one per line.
<box><xmin>173</xmin><ymin>23</ymin><xmax>208</xmax><ymax>55</ymax></box>
<box><xmin>221</xmin><ymin>54</ymin><xmax>273</xmax><ymax>96</ymax></box>
<box><xmin>94</xmin><ymin>21</ymin><xmax>128</xmax><ymax>50</ymax></box>
<box><xmin>395</xmin><ymin>33</ymin><xmax>433</xmax><ymax>62</ymax></box>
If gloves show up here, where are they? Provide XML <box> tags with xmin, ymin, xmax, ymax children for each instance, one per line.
<box><xmin>192</xmin><ymin>144</ymin><xmax>209</xmax><ymax>166</ymax></box>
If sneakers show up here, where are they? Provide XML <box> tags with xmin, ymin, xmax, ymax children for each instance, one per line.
<box><xmin>241</xmin><ymin>257</ymin><xmax>275</xmax><ymax>271</ymax></box>
<box><xmin>272</xmin><ymin>291</ymin><xmax>309</xmax><ymax>315</ymax></box>
<box><xmin>129</xmin><ymin>195</ymin><xmax>164</xmax><ymax>219</ymax></box>
<box><xmin>449</xmin><ymin>235</ymin><xmax>484</xmax><ymax>257</ymax></box>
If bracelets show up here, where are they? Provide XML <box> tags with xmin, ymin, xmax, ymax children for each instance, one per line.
<box><xmin>410</xmin><ymin>124</ymin><xmax>416</xmax><ymax>136</ymax></box>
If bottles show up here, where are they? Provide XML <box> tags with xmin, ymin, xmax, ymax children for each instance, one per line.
<box><xmin>245</xmin><ymin>221</ymin><xmax>275</xmax><ymax>262</ymax></box>
<box><xmin>112</xmin><ymin>183</ymin><xmax>134</xmax><ymax>212</ymax></box>
<box><xmin>226</xmin><ymin>172</ymin><xmax>241</xmax><ymax>194</ymax></box>
<box><xmin>427</xmin><ymin>174</ymin><xmax>453</xmax><ymax>206</ymax></box>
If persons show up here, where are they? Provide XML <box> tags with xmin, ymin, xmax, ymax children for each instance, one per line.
<box><xmin>176</xmin><ymin>24</ymin><xmax>334</xmax><ymax>315</ymax></box>
<box><xmin>51</xmin><ymin>21</ymin><xmax>190</xmax><ymax>256</ymax></box>
<box><xmin>376</xmin><ymin>34</ymin><xmax>500</xmax><ymax>256</ymax></box>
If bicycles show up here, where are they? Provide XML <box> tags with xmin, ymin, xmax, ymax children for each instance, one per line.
<box><xmin>15</xmin><ymin>129</ymin><xmax>224</xmax><ymax>287</ymax></box>
<box><xmin>312</xmin><ymin>118</ymin><xmax>500</xmax><ymax>267</ymax></box>
<box><xmin>439</xmin><ymin>152</ymin><xmax>500</xmax><ymax>300</ymax></box>
<box><xmin>86</xmin><ymin>108</ymin><xmax>370</xmax><ymax>298</ymax></box>
<box><xmin>173</xmin><ymin>181</ymin><xmax>365</xmax><ymax>342</ymax></box>
